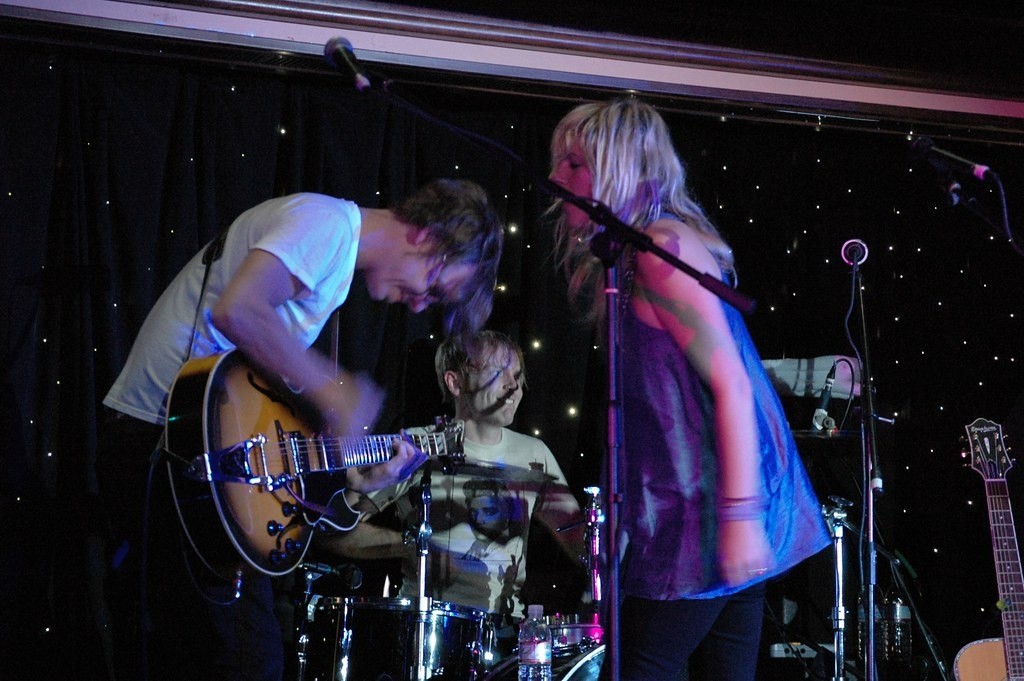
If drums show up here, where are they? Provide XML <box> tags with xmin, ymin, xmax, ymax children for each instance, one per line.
<box><xmin>830</xmin><ymin>618</ymin><xmax>948</xmax><ymax>681</ymax></box>
<box><xmin>293</xmin><ymin>592</ymin><xmax>491</xmax><ymax>680</ymax></box>
<box><xmin>483</xmin><ymin>641</ymin><xmax>712</xmax><ymax>681</ymax></box>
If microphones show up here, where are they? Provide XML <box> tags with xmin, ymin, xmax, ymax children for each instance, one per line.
<box><xmin>841</xmin><ymin>239</ymin><xmax>869</xmax><ymax>266</ymax></box>
<box><xmin>810</xmin><ymin>365</ymin><xmax>837</xmax><ymax>439</ymax></box>
<box><xmin>323</xmin><ymin>36</ymin><xmax>370</xmax><ymax>92</ymax></box>
<box><xmin>269</xmin><ymin>549</ymin><xmax>362</xmax><ymax>590</ymax></box>
<box><xmin>908</xmin><ymin>137</ymin><xmax>996</xmax><ymax>184</ymax></box>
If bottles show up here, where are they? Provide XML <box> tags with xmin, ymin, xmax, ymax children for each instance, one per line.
<box><xmin>857</xmin><ymin>577</ymin><xmax>885</xmax><ymax>660</ymax></box>
<box><xmin>553</xmin><ymin>613</ymin><xmax>564</xmax><ymax>624</ymax></box>
<box><xmin>885</xmin><ymin>575</ymin><xmax>912</xmax><ymax>660</ymax></box>
<box><xmin>519</xmin><ymin>605</ymin><xmax>552</xmax><ymax>681</ymax></box>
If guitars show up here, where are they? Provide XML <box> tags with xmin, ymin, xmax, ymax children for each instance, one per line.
<box><xmin>957</xmin><ymin>416</ymin><xmax>1023</xmax><ymax>680</ymax></box>
<box><xmin>161</xmin><ymin>346</ymin><xmax>467</xmax><ymax>584</ymax></box>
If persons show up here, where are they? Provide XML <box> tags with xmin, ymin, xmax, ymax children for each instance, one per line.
<box><xmin>321</xmin><ymin>329</ymin><xmax>594</xmax><ymax>681</ymax></box>
<box><xmin>99</xmin><ymin>178</ymin><xmax>506</xmax><ymax>680</ymax></box>
<box><xmin>548</xmin><ymin>100</ymin><xmax>784</xmax><ymax>681</ymax></box>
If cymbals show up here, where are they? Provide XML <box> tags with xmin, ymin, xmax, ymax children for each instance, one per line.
<box><xmin>792</xmin><ymin>427</ymin><xmax>865</xmax><ymax>441</ymax></box>
<box><xmin>417</xmin><ymin>456</ymin><xmax>560</xmax><ymax>484</ymax></box>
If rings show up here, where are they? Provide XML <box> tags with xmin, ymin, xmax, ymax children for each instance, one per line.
<box><xmin>748</xmin><ymin>568</ymin><xmax>770</xmax><ymax>577</ymax></box>
<box><xmin>362</xmin><ymin>425</ymin><xmax>370</xmax><ymax>431</ymax></box>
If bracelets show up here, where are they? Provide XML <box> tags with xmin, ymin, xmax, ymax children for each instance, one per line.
<box><xmin>715</xmin><ymin>495</ymin><xmax>764</xmax><ymax>522</ymax></box>
